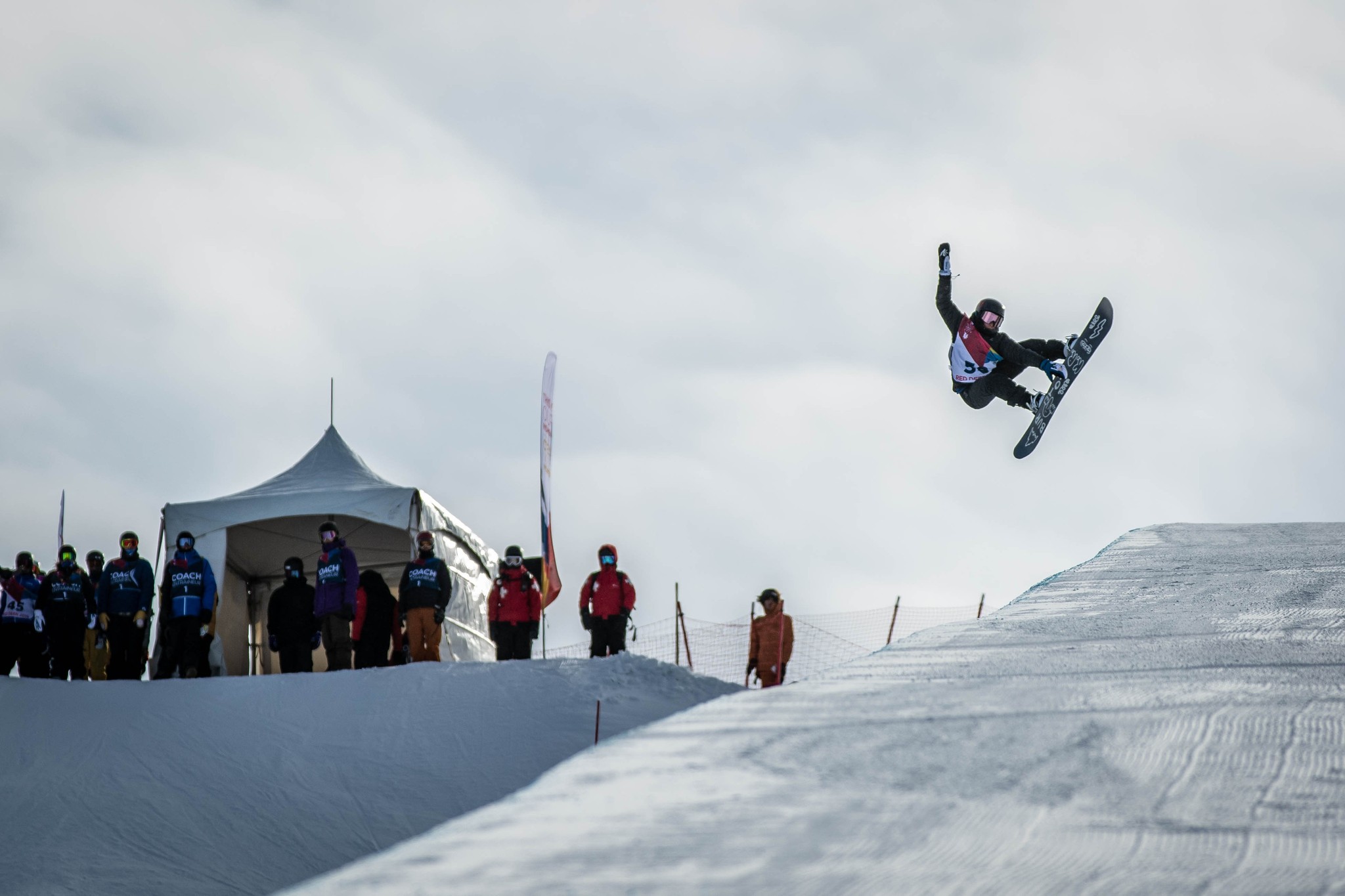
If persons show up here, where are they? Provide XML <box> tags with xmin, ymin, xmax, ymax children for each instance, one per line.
<box><xmin>153</xmin><ymin>531</ymin><xmax>218</xmax><ymax>678</ymax></box>
<box><xmin>747</xmin><ymin>589</ymin><xmax>794</xmax><ymax>688</ymax></box>
<box><xmin>488</xmin><ymin>546</ymin><xmax>542</xmax><ymax>661</ymax></box>
<box><xmin>313</xmin><ymin>521</ymin><xmax>359</xmax><ymax>674</ymax></box>
<box><xmin>350</xmin><ymin>569</ymin><xmax>402</xmax><ymax>669</ymax></box>
<box><xmin>34</xmin><ymin>545</ymin><xmax>98</xmax><ymax>680</ymax></box>
<box><xmin>81</xmin><ymin>550</ymin><xmax>110</xmax><ymax>682</ymax></box>
<box><xmin>936</xmin><ymin>243</ymin><xmax>1079</xmax><ymax>416</ymax></box>
<box><xmin>97</xmin><ymin>532</ymin><xmax>153</xmax><ymax>682</ymax></box>
<box><xmin>579</xmin><ymin>544</ymin><xmax>636</xmax><ymax>659</ymax></box>
<box><xmin>399</xmin><ymin>531</ymin><xmax>451</xmax><ymax>663</ymax></box>
<box><xmin>267</xmin><ymin>556</ymin><xmax>321</xmax><ymax>674</ymax></box>
<box><xmin>0</xmin><ymin>552</ymin><xmax>53</xmax><ymax>679</ymax></box>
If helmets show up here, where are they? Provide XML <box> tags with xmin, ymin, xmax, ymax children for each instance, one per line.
<box><xmin>16</xmin><ymin>532</ymin><xmax>194</xmax><ymax>568</ymax></box>
<box><xmin>506</xmin><ymin>545</ymin><xmax>523</xmax><ymax>558</ymax></box>
<box><xmin>761</xmin><ymin>590</ymin><xmax>778</xmax><ymax>603</ymax></box>
<box><xmin>284</xmin><ymin>556</ymin><xmax>303</xmax><ymax>571</ymax></box>
<box><xmin>976</xmin><ymin>298</ymin><xmax>1005</xmax><ymax>318</ymax></box>
<box><xmin>416</xmin><ymin>531</ymin><xmax>432</xmax><ymax>542</ymax></box>
<box><xmin>319</xmin><ymin>521</ymin><xmax>339</xmax><ymax>535</ymax></box>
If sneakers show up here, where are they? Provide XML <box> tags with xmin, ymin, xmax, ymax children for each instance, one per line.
<box><xmin>1024</xmin><ymin>392</ymin><xmax>1045</xmax><ymax>413</ymax></box>
<box><xmin>1068</xmin><ymin>338</ymin><xmax>1078</xmax><ymax>350</ymax></box>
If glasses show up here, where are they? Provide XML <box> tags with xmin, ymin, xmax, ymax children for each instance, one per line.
<box><xmin>601</xmin><ymin>555</ymin><xmax>614</xmax><ymax>564</ymax></box>
<box><xmin>287</xmin><ymin>570</ymin><xmax>300</xmax><ymax>578</ymax></box>
<box><xmin>319</xmin><ymin>530</ymin><xmax>336</xmax><ymax>541</ymax></box>
<box><xmin>418</xmin><ymin>538</ymin><xmax>433</xmax><ymax>548</ymax></box>
<box><xmin>765</xmin><ymin>591</ymin><xmax>777</xmax><ymax>597</ymax></box>
<box><xmin>981</xmin><ymin>310</ymin><xmax>1004</xmax><ymax>328</ymax></box>
<box><xmin>505</xmin><ymin>556</ymin><xmax>522</xmax><ymax>567</ymax></box>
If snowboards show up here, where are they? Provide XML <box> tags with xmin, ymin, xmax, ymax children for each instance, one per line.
<box><xmin>1012</xmin><ymin>296</ymin><xmax>1113</xmax><ymax>460</ymax></box>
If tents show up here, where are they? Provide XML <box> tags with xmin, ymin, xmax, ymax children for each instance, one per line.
<box><xmin>149</xmin><ymin>425</ymin><xmax>498</xmax><ymax>666</ymax></box>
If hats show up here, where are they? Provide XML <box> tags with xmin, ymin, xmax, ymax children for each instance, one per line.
<box><xmin>600</xmin><ymin>548</ymin><xmax>613</xmax><ymax>555</ymax></box>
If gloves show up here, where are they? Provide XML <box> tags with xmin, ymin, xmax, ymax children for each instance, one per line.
<box><xmin>939</xmin><ymin>243</ymin><xmax>951</xmax><ymax>276</ymax></box>
<box><xmin>1041</xmin><ymin>359</ymin><xmax>1068</xmax><ymax>382</ymax></box>
<box><xmin>530</xmin><ymin>621</ymin><xmax>538</xmax><ymax>639</ymax></box>
<box><xmin>33</xmin><ymin>609</ymin><xmax>208</xmax><ymax>633</ymax></box>
<box><xmin>582</xmin><ymin>607</ymin><xmax>593</xmax><ymax>629</ymax></box>
<box><xmin>490</xmin><ymin>621</ymin><xmax>497</xmax><ymax>641</ymax></box>
<box><xmin>748</xmin><ymin>659</ymin><xmax>758</xmax><ymax>675</ymax></box>
<box><xmin>620</xmin><ymin>607</ymin><xmax>630</xmax><ymax>628</ymax></box>
<box><xmin>781</xmin><ymin>663</ymin><xmax>786</xmax><ymax>676</ymax></box>
<box><xmin>269</xmin><ymin>604</ymin><xmax>445</xmax><ymax>652</ymax></box>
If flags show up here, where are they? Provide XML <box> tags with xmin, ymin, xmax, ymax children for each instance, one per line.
<box><xmin>539</xmin><ymin>352</ymin><xmax>562</xmax><ymax>610</ymax></box>
